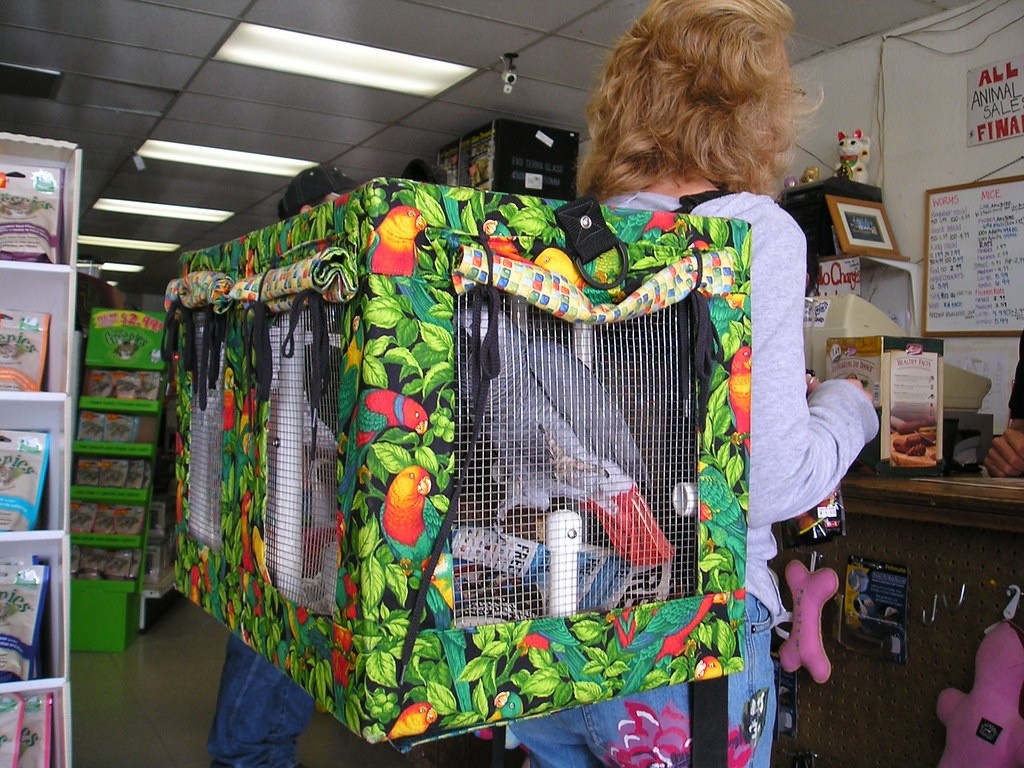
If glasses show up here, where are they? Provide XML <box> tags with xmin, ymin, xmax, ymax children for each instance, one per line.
<box><xmin>784</xmin><ymin>88</ymin><xmax>806</xmax><ymax>98</ymax></box>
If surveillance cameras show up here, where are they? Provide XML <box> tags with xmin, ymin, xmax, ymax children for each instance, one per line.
<box><xmin>501</xmin><ymin>69</ymin><xmax>517</xmax><ymax>84</ymax></box>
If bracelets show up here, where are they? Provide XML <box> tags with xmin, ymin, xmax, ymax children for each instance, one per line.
<box><xmin>807</xmin><ymin>376</ymin><xmax>816</xmax><ymax>386</ymax></box>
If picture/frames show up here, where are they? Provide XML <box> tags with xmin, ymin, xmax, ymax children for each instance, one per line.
<box><xmin>825</xmin><ymin>193</ymin><xmax>902</xmax><ymax>256</ymax></box>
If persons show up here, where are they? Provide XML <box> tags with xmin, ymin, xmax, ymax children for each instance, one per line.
<box><xmin>574</xmin><ymin>0</ymin><xmax>881</xmax><ymax>768</ymax></box>
<box><xmin>982</xmin><ymin>327</ymin><xmax>1024</xmax><ymax>476</ymax></box>
<box><xmin>205</xmin><ymin>164</ymin><xmax>374</xmax><ymax>767</ymax></box>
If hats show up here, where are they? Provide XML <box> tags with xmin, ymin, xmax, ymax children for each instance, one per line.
<box><xmin>284</xmin><ymin>167</ymin><xmax>357</xmax><ymax>210</ymax></box>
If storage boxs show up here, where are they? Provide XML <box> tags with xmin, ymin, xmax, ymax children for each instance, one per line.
<box><xmin>436</xmin><ymin>137</ymin><xmax>461</xmax><ymax>187</ymax></box>
<box><xmin>459</xmin><ymin>118</ymin><xmax>581</xmax><ymax>202</ymax></box>
<box><xmin>170</xmin><ymin>175</ymin><xmax>754</xmax><ymax>751</ymax></box>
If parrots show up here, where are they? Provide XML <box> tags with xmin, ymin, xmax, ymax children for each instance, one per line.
<box><xmin>461</xmin><ymin>302</ymin><xmax>677</xmax><ymax>563</ymax></box>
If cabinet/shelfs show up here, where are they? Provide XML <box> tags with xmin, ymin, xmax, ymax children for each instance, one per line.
<box><xmin>0</xmin><ymin>131</ymin><xmax>84</xmax><ymax>768</ymax></box>
<box><xmin>71</xmin><ymin>307</ymin><xmax>174</xmax><ymax>652</ymax></box>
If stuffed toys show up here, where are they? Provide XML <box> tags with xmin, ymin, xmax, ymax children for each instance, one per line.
<box><xmin>932</xmin><ymin>619</ymin><xmax>1024</xmax><ymax>768</ymax></box>
